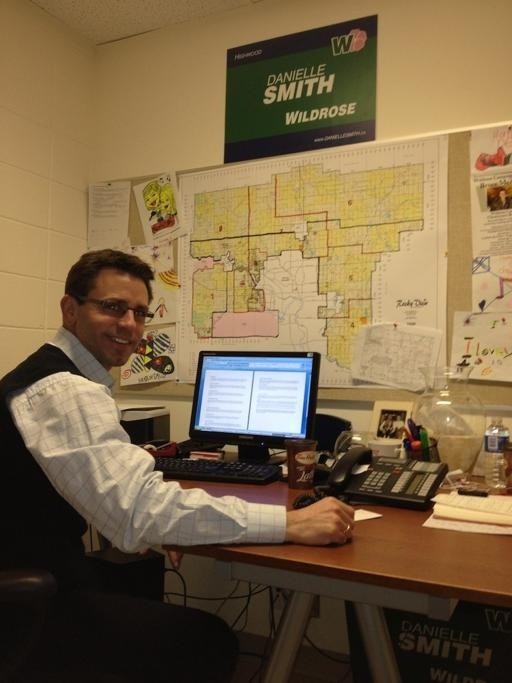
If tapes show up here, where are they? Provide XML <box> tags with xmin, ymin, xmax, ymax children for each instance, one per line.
<box><xmin>143</xmin><ymin>444</ymin><xmax>158</xmax><ymax>451</ymax></box>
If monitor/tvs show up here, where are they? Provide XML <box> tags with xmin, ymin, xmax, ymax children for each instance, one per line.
<box><xmin>189</xmin><ymin>350</ymin><xmax>321</xmax><ymax>464</ymax></box>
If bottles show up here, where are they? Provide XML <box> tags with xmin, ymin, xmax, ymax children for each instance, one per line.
<box><xmin>482</xmin><ymin>415</ymin><xmax>511</xmax><ymax>489</ymax></box>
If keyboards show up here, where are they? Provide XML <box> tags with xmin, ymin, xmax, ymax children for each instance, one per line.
<box><xmin>155</xmin><ymin>455</ymin><xmax>284</xmax><ymax>486</ymax></box>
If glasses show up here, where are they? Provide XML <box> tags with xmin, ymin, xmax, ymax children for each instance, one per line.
<box><xmin>78</xmin><ymin>296</ymin><xmax>154</xmax><ymax>324</ymax></box>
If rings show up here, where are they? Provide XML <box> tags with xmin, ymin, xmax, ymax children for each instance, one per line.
<box><xmin>342</xmin><ymin>523</ymin><xmax>350</xmax><ymax>533</ymax></box>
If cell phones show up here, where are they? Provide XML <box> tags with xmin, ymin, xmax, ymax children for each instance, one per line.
<box><xmin>147</xmin><ymin>439</ymin><xmax>173</xmax><ymax>451</ymax></box>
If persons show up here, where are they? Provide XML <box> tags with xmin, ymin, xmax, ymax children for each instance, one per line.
<box><xmin>394</xmin><ymin>414</ymin><xmax>405</xmax><ymax>438</ymax></box>
<box><xmin>379</xmin><ymin>413</ymin><xmax>396</xmax><ymax>438</ymax></box>
<box><xmin>0</xmin><ymin>247</ymin><xmax>360</xmax><ymax>682</ymax></box>
<box><xmin>489</xmin><ymin>187</ymin><xmax>512</xmax><ymax>210</ymax></box>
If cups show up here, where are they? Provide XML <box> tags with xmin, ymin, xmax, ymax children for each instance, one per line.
<box><xmin>283</xmin><ymin>439</ymin><xmax>318</xmax><ymax>490</ymax></box>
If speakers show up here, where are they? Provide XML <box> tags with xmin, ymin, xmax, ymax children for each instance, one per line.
<box><xmin>314</xmin><ymin>411</ymin><xmax>353</xmax><ymax>464</ymax></box>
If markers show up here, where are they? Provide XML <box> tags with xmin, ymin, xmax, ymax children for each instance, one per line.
<box><xmin>401</xmin><ymin>418</ymin><xmax>434</xmax><ymax>463</ymax></box>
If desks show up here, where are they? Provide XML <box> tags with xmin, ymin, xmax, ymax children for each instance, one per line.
<box><xmin>161</xmin><ymin>472</ymin><xmax>512</xmax><ymax>682</ymax></box>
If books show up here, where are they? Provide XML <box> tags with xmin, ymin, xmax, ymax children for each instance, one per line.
<box><xmin>429</xmin><ymin>487</ymin><xmax>511</xmax><ymax>527</ymax></box>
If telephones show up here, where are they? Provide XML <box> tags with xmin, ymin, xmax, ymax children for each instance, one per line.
<box><xmin>329</xmin><ymin>446</ymin><xmax>450</xmax><ymax>511</ymax></box>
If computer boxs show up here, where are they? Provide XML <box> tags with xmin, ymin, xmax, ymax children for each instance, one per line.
<box><xmin>79</xmin><ymin>548</ymin><xmax>166</xmax><ymax>604</ymax></box>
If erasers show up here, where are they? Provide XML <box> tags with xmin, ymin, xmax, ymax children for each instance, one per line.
<box><xmin>458</xmin><ymin>487</ymin><xmax>490</xmax><ymax>496</ymax></box>
